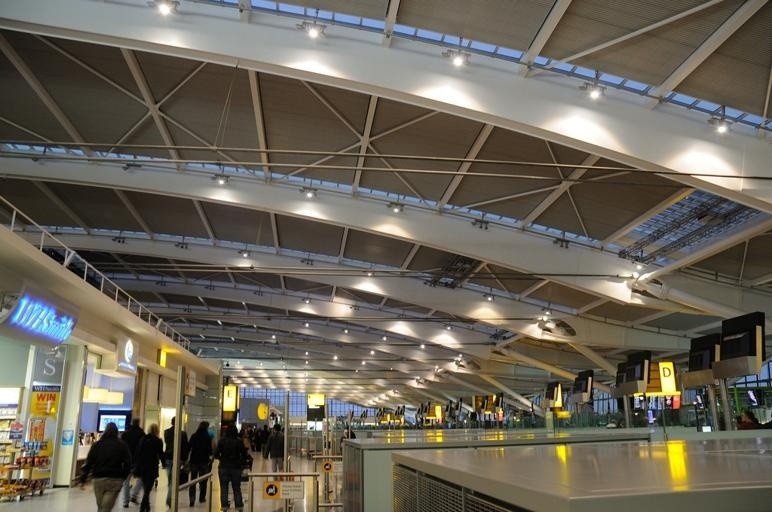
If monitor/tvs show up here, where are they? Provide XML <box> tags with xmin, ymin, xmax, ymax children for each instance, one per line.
<box><xmin>688</xmin><ymin>334</ymin><xmax>721</xmax><ymax>371</ymax></box>
<box><xmin>574</xmin><ymin>370</ymin><xmax>594</xmax><ymax>393</ymax></box>
<box><xmin>616</xmin><ymin>350</ymin><xmax>651</xmax><ymax>384</ymax></box>
<box><xmin>361</xmin><ymin>393</ymin><xmax>503</xmax><ymax>417</ymax></box>
<box><xmin>545</xmin><ymin>382</ymin><xmax>559</xmax><ymax>400</ymax></box>
<box><xmin>747</xmin><ymin>389</ymin><xmax>764</xmax><ymax>405</ymax></box>
<box><xmin>720</xmin><ymin>312</ymin><xmax>766</xmax><ymax>361</ymax></box>
<box><xmin>96</xmin><ymin>410</ymin><xmax>132</xmax><ymax>433</ymax></box>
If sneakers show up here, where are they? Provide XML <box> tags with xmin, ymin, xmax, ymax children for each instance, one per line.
<box><xmin>123</xmin><ymin>495</ymin><xmax>141</xmax><ymax>507</ymax></box>
<box><xmin>189</xmin><ymin>497</ymin><xmax>206</xmax><ymax>507</ymax></box>
<box><xmin>221</xmin><ymin>504</ymin><xmax>245</xmax><ymax>512</ymax></box>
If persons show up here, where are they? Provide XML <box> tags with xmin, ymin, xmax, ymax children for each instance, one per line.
<box><xmin>737</xmin><ymin>410</ymin><xmax>763</xmax><ymax>428</ymax></box>
<box><xmin>409</xmin><ymin>446</ymin><xmax>418</xmax><ymax>453</ymax></box>
<box><xmin>117</xmin><ymin>418</ymin><xmax>147</xmax><ymax>507</ymax></box>
<box><xmin>134</xmin><ymin>421</ymin><xmax>166</xmax><ymax>512</ymax></box>
<box><xmin>347</xmin><ymin>424</ymin><xmax>357</xmax><ymax>438</ymax></box>
<box><xmin>80</xmin><ymin>422</ymin><xmax>135</xmax><ymax>511</ymax></box>
<box><xmin>214</xmin><ymin>422</ymin><xmax>248</xmax><ymax>512</ymax></box>
<box><xmin>241</xmin><ymin>423</ymin><xmax>284</xmax><ymax>479</ymax></box>
<box><xmin>163</xmin><ymin>416</ymin><xmax>188</xmax><ymax>508</ymax></box>
<box><xmin>182</xmin><ymin>421</ymin><xmax>218</xmax><ymax>507</ymax></box>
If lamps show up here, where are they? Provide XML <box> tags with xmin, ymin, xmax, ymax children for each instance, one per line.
<box><xmin>302</xmin><ymin>20</ymin><xmax>324</xmax><ymax>38</ymax></box>
<box><xmin>211</xmin><ymin>165</ymin><xmax>229</xmax><ymax>185</ymax></box>
<box><xmin>114</xmin><ymin>231</ymin><xmax>567</xmax><ymax>332</ymax></box>
<box><xmin>82</xmin><ymin>363</ymin><xmax>109</xmax><ymax>403</ymax></box>
<box><xmin>580</xmin><ymin>81</ymin><xmax>607</xmax><ymax>100</ymax></box>
<box><xmin>471</xmin><ymin>210</ymin><xmax>490</xmax><ymax>230</ymax></box>
<box><xmin>707</xmin><ymin>116</ymin><xmax>731</xmax><ymax>135</ymax></box>
<box><xmin>99</xmin><ymin>375</ymin><xmax>124</xmax><ymax>405</ymax></box>
<box><xmin>445</xmin><ymin>47</ymin><xmax>470</xmax><ymax>68</ymax></box>
<box><xmin>33</xmin><ymin>147</ymin><xmax>48</xmax><ymax>165</ymax></box>
<box><xmin>122</xmin><ymin>155</ymin><xmax>142</xmax><ymax>171</ymax></box>
<box><xmin>154</xmin><ymin>0</ymin><xmax>178</xmax><ymax>16</ymax></box>
<box><xmin>386</xmin><ymin>193</ymin><xmax>404</xmax><ymax>214</ymax></box>
<box><xmin>299</xmin><ymin>176</ymin><xmax>319</xmax><ymax>198</ymax></box>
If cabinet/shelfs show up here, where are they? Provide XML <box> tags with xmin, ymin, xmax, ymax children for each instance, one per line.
<box><xmin>7</xmin><ymin>447</ymin><xmax>52</xmax><ymax>502</ymax></box>
<box><xmin>1</xmin><ymin>416</ymin><xmax>17</xmax><ymax>492</ymax></box>
